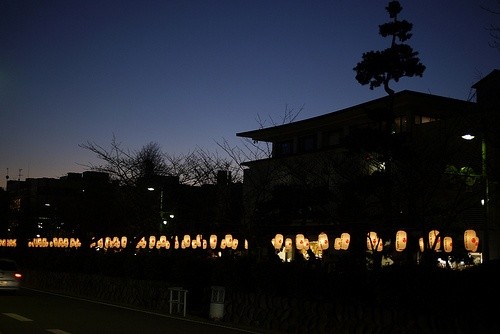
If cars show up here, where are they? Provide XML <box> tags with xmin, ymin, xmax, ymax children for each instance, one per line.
<box><xmin>0</xmin><ymin>258</ymin><xmax>23</xmax><ymax>289</ymax></box>
